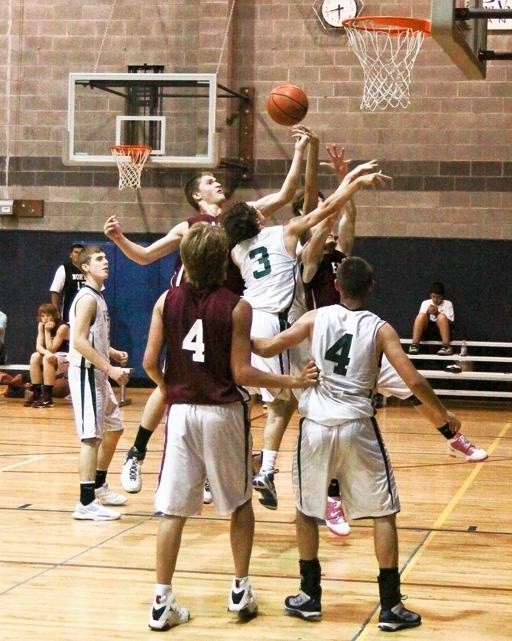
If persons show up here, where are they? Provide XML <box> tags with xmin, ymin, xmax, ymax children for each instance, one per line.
<box><xmin>0</xmin><ymin>311</ymin><xmax>9</xmax><ymax>365</ymax></box>
<box><xmin>67</xmin><ymin>245</ymin><xmax>129</xmax><ymax>524</ymax></box>
<box><xmin>250</xmin><ymin>254</ymin><xmax>463</xmax><ymax>634</ymax></box>
<box><xmin>104</xmin><ymin>123</ymin><xmax>493</xmax><ymax>539</ymax></box>
<box><xmin>23</xmin><ymin>303</ymin><xmax>70</xmax><ymax>410</ymax></box>
<box><xmin>142</xmin><ymin>219</ymin><xmax>322</xmax><ymax>634</ymax></box>
<box><xmin>49</xmin><ymin>241</ymin><xmax>106</xmax><ymax>356</ymax></box>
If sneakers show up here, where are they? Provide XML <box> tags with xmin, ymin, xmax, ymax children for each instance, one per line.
<box><xmin>408</xmin><ymin>343</ymin><xmax>419</xmax><ymax>354</ymax></box>
<box><xmin>71</xmin><ymin>498</ymin><xmax>120</xmax><ymax>521</ymax></box>
<box><xmin>227</xmin><ymin>580</ymin><xmax>258</xmax><ymax>618</ymax></box>
<box><xmin>437</xmin><ymin>344</ymin><xmax>454</xmax><ymax>355</ymax></box>
<box><xmin>378</xmin><ymin>602</ymin><xmax>420</xmax><ymax>631</ymax></box>
<box><xmin>149</xmin><ymin>595</ymin><xmax>190</xmax><ymax>631</ymax></box>
<box><xmin>120</xmin><ymin>447</ymin><xmax>147</xmax><ymax>494</ymax></box>
<box><xmin>324</xmin><ymin>495</ymin><xmax>351</xmax><ymax>535</ymax></box>
<box><xmin>203</xmin><ymin>479</ymin><xmax>212</xmax><ymax>504</ymax></box>
<box><xmin>447</xmin><ymin>433</ymin><xmax>488</xmax><ymax>462</ymax></box>
<box><xmin>284</xmin><ymin>589</ymin><xmax>322</xmax><ymax>620</ymax></box>
<box><xmin>253</xmin><ymin>466</ymin><xmax>279</xmax><ymax>510</ymax></box>
<box><xmin>95</xmin><ymin>482</ymin><xmax>127</xmax><ymax>506</ymax></box>
<box><xmin>24</xmin><ymin>394</ymin><xmax>54</xmax><ymax>408</ymax></box>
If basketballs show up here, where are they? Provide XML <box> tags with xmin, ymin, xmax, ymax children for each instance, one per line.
<box><xmin>267</xmin><ymin>83</ymin><xmax>308</xmax><ymax>126</ymax></box>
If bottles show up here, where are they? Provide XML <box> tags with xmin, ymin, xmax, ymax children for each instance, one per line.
<box><xmin>460</xmin><ymin>340</ymin><xmax>467</xmax><ymax>356</ymax></box>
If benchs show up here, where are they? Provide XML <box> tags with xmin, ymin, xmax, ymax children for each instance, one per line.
<box><xmin>396</xmin><ymin>339</ymin><xmax>512</xmax><ymax>398</ymax></box>
<box><xmin>0</xmin><ymin>365</ymin><xmax>135</xmax><ymax>407</ymax></box>
